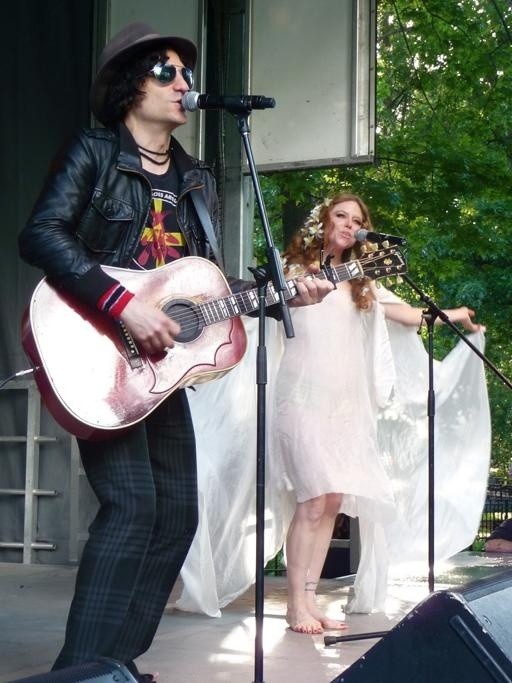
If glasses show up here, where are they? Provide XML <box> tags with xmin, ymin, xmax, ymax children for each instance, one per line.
<box><xmin>129</xmin><ymin>60</ymin><xmax>198</xmax><ymax>88</ymax></box>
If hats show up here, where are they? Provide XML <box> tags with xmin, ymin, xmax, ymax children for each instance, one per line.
<box><xmin>87</xmin><ymin>19</ymin><xmax>200</xmax><ymax>129</ymax></box>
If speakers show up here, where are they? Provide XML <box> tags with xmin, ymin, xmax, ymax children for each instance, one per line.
<box><xmin>11</xmin><ymin>653</ymin><xmax>139</xmax><ymax>683</ymax></box>
<box><xmin>330</xmin><ymin>566</ymin><xmax>512</xmax><ymax>683</ymax></box>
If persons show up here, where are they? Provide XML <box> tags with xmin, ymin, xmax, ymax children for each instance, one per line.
<box><xmin>18</xmin><ymin>20</ymin><xmax>338</xmax><ymax>683</ymax></box>
<box><xmin>179</xmin><ymin>191</ymin><xmax>492</xmax><ymax>638</ymax></box>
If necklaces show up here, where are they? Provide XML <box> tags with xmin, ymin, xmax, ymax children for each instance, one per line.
<box><xmin>136</xmin><ymin>142</ymin><xmax>172</xmax><ymax>165</ymax></box>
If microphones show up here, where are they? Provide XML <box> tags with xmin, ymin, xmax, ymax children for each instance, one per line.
<box><xmin>354</xmin><ymin>227</ymin><xmax>410</xmax><ymax>247</ymax></box>
<box><xmin>178</xmin><ymin>90</ymin><xmax>274</xmax><ymax>110</ymax></box>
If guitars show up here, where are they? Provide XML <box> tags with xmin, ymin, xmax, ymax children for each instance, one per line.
<box><xmin>21</xmin><ymin>240</ymin><xmax>408</xmax><ymax>441</ymax></box>
